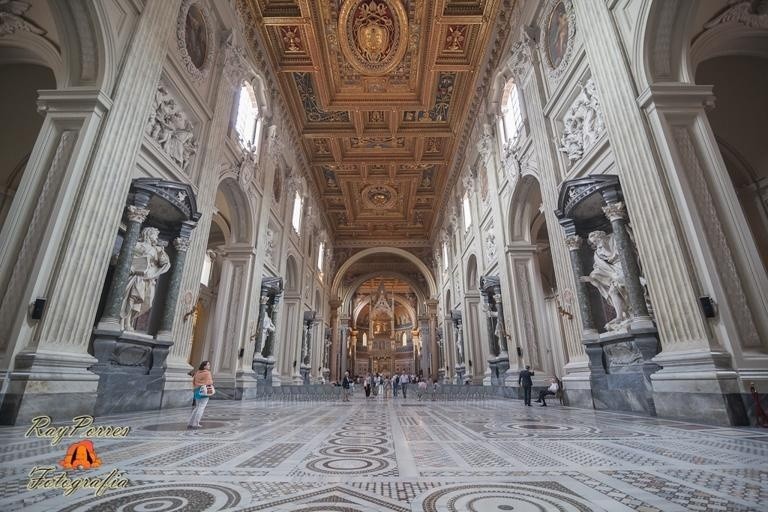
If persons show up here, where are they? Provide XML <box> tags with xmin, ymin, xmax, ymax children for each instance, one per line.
<box><xmin>186</xmin><ymin>360</ymin><xmax>214</xmax><ymax>430</ymax></box>
<box><xmin>483</xmin><ymin>293</ymin><xmax>502</xmax><ymax>350</ymax></box>
<box><xmin>342</xmin><ymin>369</ymin><xmax>438</xmax><ymax>401</ymax></box>
<box><xmin>261</xmin><ymin>306</ymin><xmax>275</xmax><ymax>349</ymax></box>
<box><xmin>320</xmin><ymin>378</ymin><xmax>323</xmax><ymax>385</ymax></box>
<box><xmin>578</xmin><ymin>231</ymin><xmax>650</xmax><ymax>325</ymax></box>
<box><xmin>455</xmin><ymin>323</ymin><xmax>463</xmax><ymax>360</ymax></box>
<box><xmin>519</xmin><ymin>366</ymin><xmax>535</xmax><ymax>406</ymax></box>
<box><xmin>115</xmin><ymin>226</ymin><xmax>171</xmax><ymax>332</ymax></box>
<box><xmin>534</xmin><ymin>376</ymin><xmax>559</xmax><ymax>406</ymax></box>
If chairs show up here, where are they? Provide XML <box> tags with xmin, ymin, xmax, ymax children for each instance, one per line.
<box><xmin>254</xmin><ymin>381</ymin><xmax>347</xmax><ymax>403</ymax></box>
<box><xmin>552</xmin><ymin>387</ymin><xmax>566</xmax><ymax>407</ymax></box>
<box><xmin>404</xmin><ymin>377</ymin><xmax>501</xmax><ymax>402</ymax></box>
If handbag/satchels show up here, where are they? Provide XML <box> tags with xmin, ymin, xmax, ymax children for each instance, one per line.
<box><xmin>199</xmin><ymin>385</ymin><xmax>215</xmax><ymax>396</ymax></box>
